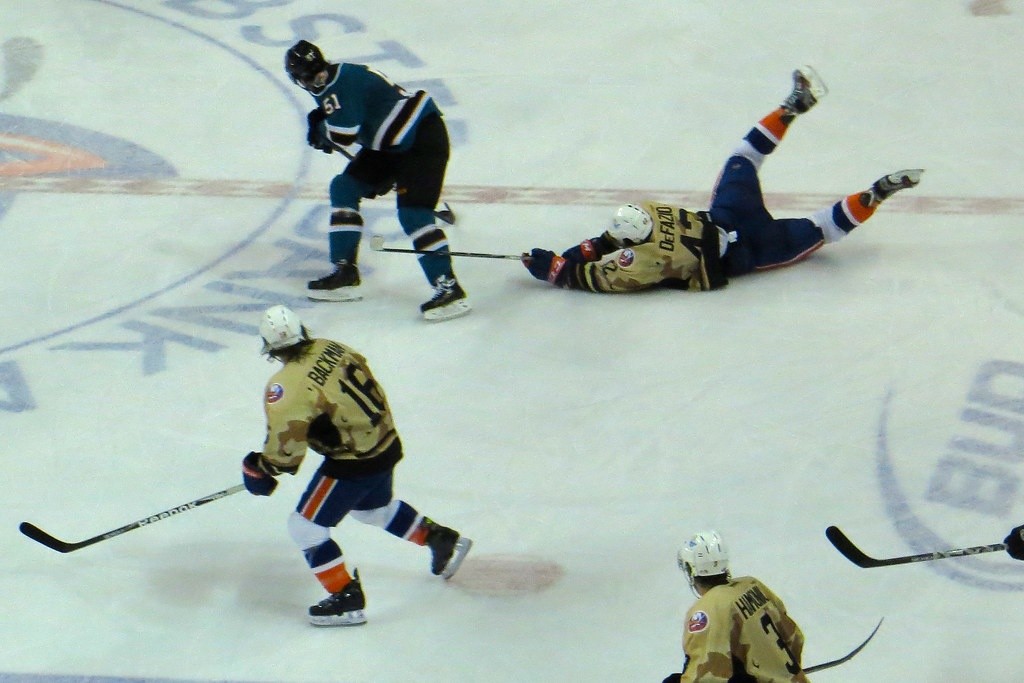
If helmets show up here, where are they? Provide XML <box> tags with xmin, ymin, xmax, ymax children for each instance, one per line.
<box><xmin>677</xmin><ymin>529</ymin><xmax>730</xmax><ymax>585</ymax></box>
<box><xmin>607</xmin><ymin>204</ymin><xmax>654</xmax><ymax>246</ymax></box>
<box><xmin>259</xmin><ymin>305</ymin><xmax>306</xmax><ymax>353</ymax></box>
<box><xmin>284</xmin><ymin>40</ymin><xmax>328</xmax><ymax>89</ymax></box>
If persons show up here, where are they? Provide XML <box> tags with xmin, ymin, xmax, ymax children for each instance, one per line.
<box><xmin>523</xmin><ymin>66</ymin><xmax>924</xmax><ymax>294</ymax></box>
<box><xmin>662</xmin><ymin>530</ymin><xmax>810</xmax><ymax>683</ymax></box>
<box><xmin>242</xmin><ymin>305</ymin><xmax>473</xmax><ymax>628</ymax></box>
<box><xmin>285</xmin><ymin>40</ymin><xmax>473</xmax><ymax>325</ymax></box>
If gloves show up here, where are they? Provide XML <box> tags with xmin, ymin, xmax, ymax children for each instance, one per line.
<box><xmin>1004</xmin><ymin>524</ymin><xmax>1024</xmax><ymax>561</ymax></box>
<box><xmin>562</xmin><ymin>238</ymin><xmax>598</xmax><ymax>263</ymax></box>
<box><xmin>522</xmin><ymin>248</ymin><xmax>569</xmax><ymax>287</ymax></box>
<box><xmin>243</xmin><ymin>451</ymin><xmax>277</xmax><ymax>496</ymax></box>
<box><xmin>306</xmin><ymin>107</ymin><xmax>334</xmax><ymax>153</ymax></box>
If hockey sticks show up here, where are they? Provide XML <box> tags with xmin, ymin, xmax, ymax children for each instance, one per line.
<box><xmin>801</xmin><ymin>617</ymin><xmax>884</xmax><ymax>675</ymax></box>
<box><xmin>19</xmin><ymin>483</ymin><xmax>248</xmax><ymax>554</ymax></box>
<box><xmin>826</xmin><ymin>526</ymin><xmax>1009</xmax><ymax>569</ymax></box>
<box><xmin>370</xmin><ymin>235</ymin><xmax>534</xmax><ymax>260</ymax></box>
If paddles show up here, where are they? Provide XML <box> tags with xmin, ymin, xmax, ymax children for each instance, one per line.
<box><xmin>329</xmin><ymin>141</ymin><xmax>455</xmax><ymax>225</ymax></box>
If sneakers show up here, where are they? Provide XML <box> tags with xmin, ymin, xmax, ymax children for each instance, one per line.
<box><xmin>420</xmin><ymin>516</ymin><xmax>471</xmax><ymax>579</ymax></box>
<box><xmin>780</xmin><ymin>64</ymin><xmax>827</xmax><ymax>125</ymax></box>
<box><xmin>305</xmin><ymin>259</ymin><xmax>363</xmax><ymax>301</ymax></box>
<box><xmin>309</xmin><ymin>569</ymin><xmax>366</xmax><ymax>626</ymax></box>
<box><xmin>419</xmin><ymin>272</ymin><xmax>472</xmax><ymax>320</ymax></box>
<box><xmin>859</xmin><ymin>169</ymin><xmax>924</xmax><ymax>207</ymax></box>
